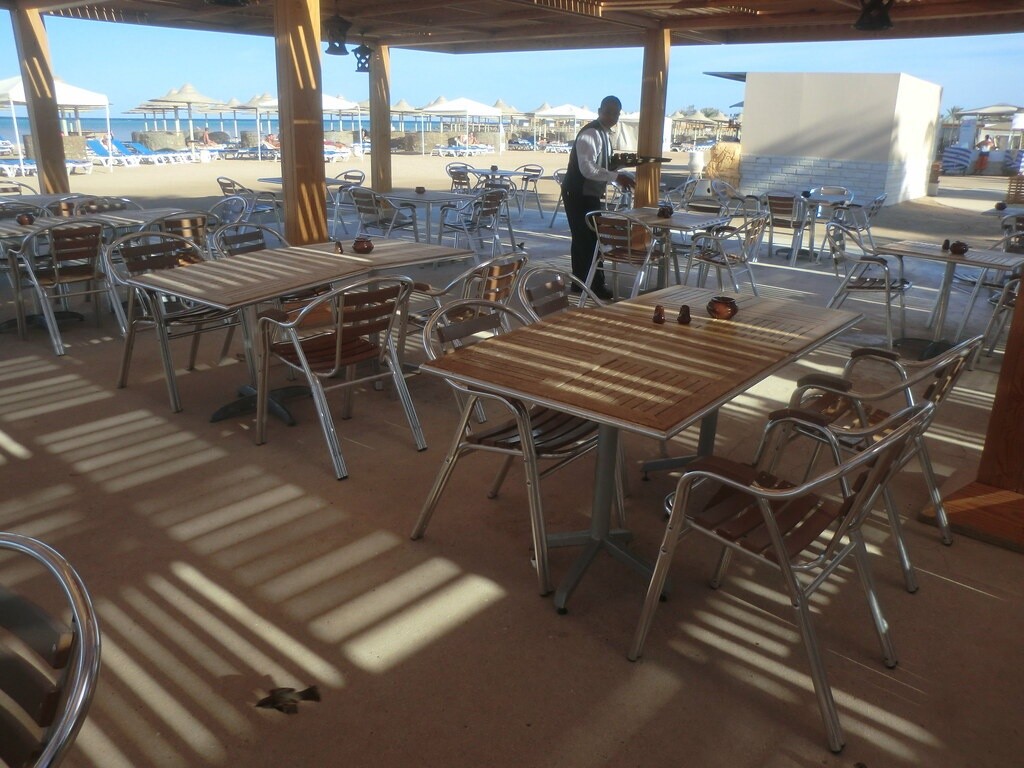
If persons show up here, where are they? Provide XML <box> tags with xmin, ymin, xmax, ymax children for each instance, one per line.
<box><xmin>266</xmin><ymin>133</ymin><xmax>279</xmax><ymax>148</ymax></box>
<box><xmin>971</xmin><ymin>134</ymin><xmax>993</xmax><ymax>174</ymax></box>
<box><xmin>102</xmin><ymin>137</ymin><xmax>111</xmax><ymax>151</ymax></box>
<box><xmin>561</xmin><ymin>96</ymin><xmax>636</xmax><ymax>300</ymax></box>
<box><xmin>202</xmin><ymin>127</ymin><xmax>212</xmax><ymax>145</ymax></box>
<box><xmin>468</xmin><ymin>132</ymin><xmax>480</xmax><ymax>145</ymax></box>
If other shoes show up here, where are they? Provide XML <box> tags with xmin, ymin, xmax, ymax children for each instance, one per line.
<box><xmin>588</xmin><ymin>284</ymin><xmax>613</xmax><ymax>299</ymax></box>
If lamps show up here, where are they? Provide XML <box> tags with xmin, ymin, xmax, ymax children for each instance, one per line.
<box><xmin>352</xmin><ymin>31</ymin><xmax>375</xmax><ymax>72</ymax></box>
<box><xmin>322</xmin><ymin>0</ymin><xmax>351</xmax><ymax>55</ymax></box>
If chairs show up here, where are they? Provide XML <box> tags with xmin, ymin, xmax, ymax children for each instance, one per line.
<box><xmin>0</xmin><ymin>139</ymin><xmax>1024</xmax><ymax>768</ymax></box>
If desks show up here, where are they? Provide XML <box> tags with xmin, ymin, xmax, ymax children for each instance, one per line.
<box><xmin>597</xmin><ymin>207</ymin><xmax>732</xmax><ymax>302</ymax></box>
<box><xmin>255</xmin><ymin>177</ymin><xmax>351</xmax><ymax>186</ymax></box>
<box><xmin>981</xmin><ymin>206</ymin><xmax>1024</xmax><ymax>252</ymax></box>
<box><xmin>282</xmin><ymin>235</ymin><xmax>476</xmax><ymax>345</ymax></box>
<box><xmin>0</xmin><ymin>192</ymin><xmax>97</xmax><ymax>213</ymax></box>
<box><xmin>0</xmin><ymin>208</ymin><xmax>204</xmax><ymax>333</ymax></box>
<box><xmin>465</xmin><ymin>168</ymin><xmax>531</xmax><ymax>188</ymax></box>
<box><xmin>873</xmin><ymin>239</ymin><xmax>1024</xmax><ymax>361</ymax></box>
<box><xmin>126</xmin><ymin>248</ymin><xmax>370</xmax><ymax>426</ymax></box>
<box><xmin>378</xmin><ymin>191</ymin><xmax>476</xmax><ymax>244</ymax></box>
<box><xmin>420</xmin><ymin>282</ymin><xmax>866</xmax><ymax>616</ymax></box>
<box><xmin>775</xmin><ymin>199</ymin><xmax>834</xmax><ymax>262</ymax></box>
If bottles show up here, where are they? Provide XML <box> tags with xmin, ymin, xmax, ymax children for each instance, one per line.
<box><xmin>15</xmin><ymin>212</ymin><xmax>36</xmax><ymax>225</ymax></box>
<box><xmin>335</xmin><ymin>241</ymin><xmax>343</xmax><ymax>254</ymax></box>
<box><xmin>352</xmin><ymin>237</ymin><xmax>374</xmax><ymax>253</ymax></box>
<box><xmin>706</xmin><ymin>296</ymin><xmax>739</xmax><ymax>319</ymax></box>
<box><xmin>653</xmin><ymin>305</ymin><xmax>665</xmax><ymax>324</ymax></box>
<box><xmin>677</xmin><ymin>305</ymin><xmax>692</xmax><ymax>325</ymax></box>
<box><xmin>942</xmin><ymin>239</ymin><xmax>949</xmax><ymax>250</ymax></box>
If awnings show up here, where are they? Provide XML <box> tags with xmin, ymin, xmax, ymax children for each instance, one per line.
<box><xmin>703</xmin><ymin>71</ymin><xmax>747</xmax><ymax>82</ymax></box>
<box><xmin>729</xmin><ymin>100</ymin><xmax>744</xmax><ymax>108</ymax></box>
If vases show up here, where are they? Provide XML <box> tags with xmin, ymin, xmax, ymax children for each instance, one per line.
<box><xmin>16</xmin><ymin>213</ymin><xmax>35</xmax><ymax>225</ymax></box>
<box><xmin>801</xmin><ymin>191</ymin><xmax>811</xmax><ymax>198</ymax></box>
<box><xmin>491</xmin><ymin>166</ymin><xmax>498</xmax><ymax>171</ymax></box>
<box><xmin>707</xmin><ymin>297</ymin><xmax>739</xmax><ymax>319</ymax></box>
<box><xmin>415</xmin><ymin>187</ymin><xmax>425</xmax><ymax>194</ymax></box>
<box><xmin>950</xmin><ymin>242</ymin><xmax>968</xmax><ymax>255</ymax></box>
<box><xmin>995</xmin><ymin>203</ymin><xmax>1007</xmax><ymax>211</ymax></box>
<box><xmin>352</xmin><ymin>237</ymin><xmax>374</xmax><ymax>253</ymax></box>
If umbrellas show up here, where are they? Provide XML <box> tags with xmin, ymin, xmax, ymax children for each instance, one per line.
<box><xmin>120</xmin><ymin>83</ymin><xmax>228</xmax><ymax>142</ymax></box>
<box><xmin>531</xmin><ymin>102</ymin><xmax>554</xmax><ymax>142</ymax></box>
<box><xmin>492</xmin><ymin>97</ymin><xmax>528</xmax><ymax>137</ymax></box>
<box><xmin>667</xmin><ymin>109</ymin><xmax>742</xmax><ymax>146</ymax></box>
<box><xmin>359</xmin><ymin>96</ymin><xmax>453</xmax><ymax>132</ymax></box>
<box><xmin>226</xmin><ymin>91</ymin><xmax>279</xmax><ymax>161</ymax></box>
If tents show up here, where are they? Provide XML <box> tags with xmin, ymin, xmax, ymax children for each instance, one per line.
<box><xmin>424</xmin><ymin>97</ymin><xmax>502</xmax><ymax>157</ymax></box>
<box><xmin>322</xmin><ymin>94</ymin><xmax>363</xmax><ymax>155</ymax></box>
<box><xmin>0</xmin><ymin>72</ymin><xmax>112</xmax><ymax>176</ymax></box>
<box><xmin>949</xmin><ymin>104</ymin><xmax>1024</xmax><ymax>149</ymax></box>
<box><xmin>535</xmin><ymin>102</ymin><xmax>603</xmax><ymax>142</ymax></box>
<box><xmin>617</xmin><ymin>110</ymin><xmax>673</xmax><ymax>152</ymax></box>
